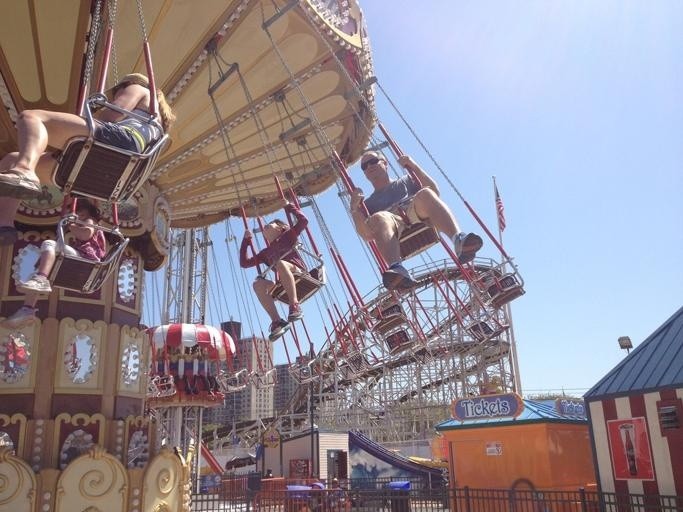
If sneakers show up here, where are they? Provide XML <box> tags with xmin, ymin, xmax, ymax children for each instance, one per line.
<box><xmin>0</xmin><ymin>306</ymin><xmax>39</xmax><ymax>330</ymax></box>
<box><xmin>0</xmin><ymin>168</ymin><xmax>42</xmax><ymax>199</ymax></box>
<box><xmin>454</xmin><ymin>232</ymin><xmax>484</xmax><ymax>264</ymax></box>
<box><xmin>382</xmin><ymin>265</ymin><xmax>415</xmax><ymax>290</ymax></box>
<box><xmin>15</xmin><ymin>276</ymin><xmax>52</xmax><ymax>294</ymax></box>
<box><xmin>268</xmin><ymin>318</ymin><xmax>291</xmax><ymax>342</ymax></box>
<box><xmin>287</xmin><ymin>303</ymin><xmax>304</xmax><ymax>322</ymax></box>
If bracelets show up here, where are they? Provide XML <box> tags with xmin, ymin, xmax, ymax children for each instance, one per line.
<box><xmin>348</xmin><ymin>204</ymin><xmax>360</xmax><ymax>214</ymax></box>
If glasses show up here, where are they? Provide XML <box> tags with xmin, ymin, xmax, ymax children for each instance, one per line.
<box><xmin>112</xmin><ymin>81</ymin><xmax>130</xmax><ymax>95</ymax></box>
<box><xmin>361</xmin><ymin>157</ymin><xmax>385</xmax><ymax>171</ymax></box>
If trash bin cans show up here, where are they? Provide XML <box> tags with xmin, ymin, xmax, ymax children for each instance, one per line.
<box><xmin>285</xmin><ymin>483</ymin><xmax>327</xmax><ymax>512</ymax></box>
<box><xmin>389</xmin><ymin>481</ymin><xmax>411</xmax><ymax>512</ymax></box>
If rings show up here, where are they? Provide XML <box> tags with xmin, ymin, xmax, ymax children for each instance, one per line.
<box><xmin>402</xmin><ymin>157</ymin><xmax>405</xmax><ymax>160</ymax></box>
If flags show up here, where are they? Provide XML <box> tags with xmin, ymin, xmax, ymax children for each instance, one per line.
<box><xmin>493</xmin><ymin>179</ymin><xmax>506</xmax><ymax>233</ymax></box>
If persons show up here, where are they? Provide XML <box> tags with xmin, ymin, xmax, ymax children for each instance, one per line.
<box><xmin>0</xmin><ymin>199</ymin><xmax>108</xmax><ymax>333</ymax></box>
<box><xmin>156</xmin><ymin>206</ymin><xmax>167</xmax><ymax>243</ymax></box>
<box><xmin>36</xmin><ymin>184</ymin><xmax>53</xmax><ymax>205</ymax></box>
<box><xmin>0</xmin><ymin>71</ymin><xmax>176</xmax><ymax>201</ymax></box>
<box><xmin>319</xmin><ymin>477</ymin><xmax>351</xmax><ymax>512</ymax></box>
<box><xmin>238</xmin><ymin>198</ymin><xmax>313</xmax><ymax>342</ymax></box>
<box><xmin>263</xmin><ymin>468</ymin><xmax>275</xmax><ymax>479</ymax></box>
<box><xmin>347</xmin><ymin>151</ymin><xmax>483</xmax><ymax>292</ymax></box>
